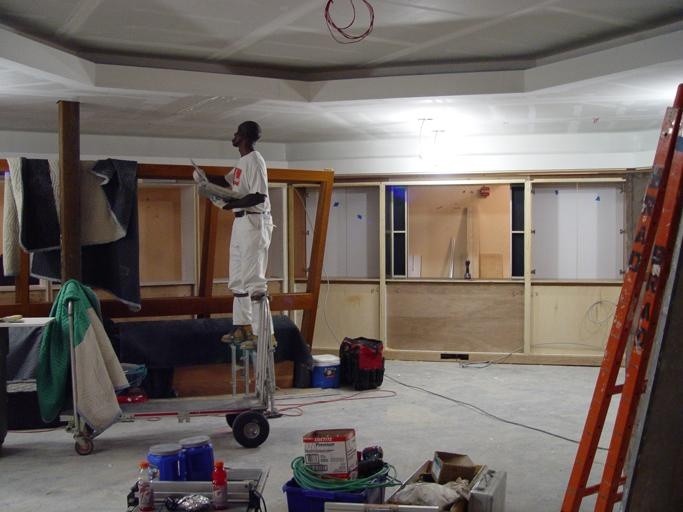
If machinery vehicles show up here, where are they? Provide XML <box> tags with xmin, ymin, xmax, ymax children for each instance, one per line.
<box><xmin>58</xmin><ymin>286</ymin><xmax>276</xmax><ymax>458</ymax></box>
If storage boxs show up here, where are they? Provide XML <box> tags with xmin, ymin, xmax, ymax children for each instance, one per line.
<box><xmin>303</xmin><ymin>427</ymin><xmax>357</xmax><ymax>480</ymax></box>
<box><xmin>281</xmin><ymin>461</ymin><xmax>389</xmax><ymax>512</ymax></box>
<box><xmin>430</xmin><ymin>451</ymin><xmax>476</xmax><ymax>480</ymax></box>
<box><xmin>308</xmin><ymin>354</ymin><xmax>340</xmax><ymax>390</ymax></box>
<box><xmin>386</xmin><ymin>460</ymin><xmax>487</xmax><ymax>512</ymax></box>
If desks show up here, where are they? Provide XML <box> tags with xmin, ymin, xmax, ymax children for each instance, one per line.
<box><xmin>0</xmin><ymin>315</ymin><xmax>56</xmax><ymax>447</ymax></box>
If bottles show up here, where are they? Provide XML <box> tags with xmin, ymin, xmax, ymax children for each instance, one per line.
<box><xmin>212</xmin><ymin>460</ymin><xmax>228</xmax><ymax>510</ymax></box>
<box><xmin>137</xmin><ymin>461</ymin><xmax>155</xmax><ymax>512</ymax></box>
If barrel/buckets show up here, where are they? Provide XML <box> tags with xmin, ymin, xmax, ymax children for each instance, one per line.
<box><xmin>146</xmin><ymin>443</ymin><xmax>187</xmax><ymax>481</ymax></box>
<box><xmin>179</xmin><ymin>435</ymin><xmax>214</xmax><ymax>481</ymax></box>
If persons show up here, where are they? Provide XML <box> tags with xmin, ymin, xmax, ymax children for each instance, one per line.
<box><xmin>193</xmin><ymin>120</ymin><xmax>278</xmax><ymax>351</ymax></box>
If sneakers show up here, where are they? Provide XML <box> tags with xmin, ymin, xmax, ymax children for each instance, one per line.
<box><xmin>241</xmin><ymin>335</ymin><xmax>277</xmax><ymax>349</ymax></box>
<box><xmin>221</xmin><ymin>325</ymin><xmax>255</xmax><ymax>343</ymax></box>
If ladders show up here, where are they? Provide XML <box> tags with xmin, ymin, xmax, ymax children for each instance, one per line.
<box><xmin>560</xmin><ymin>100</ymin><xmax>683</xmax><ymax>512</ymax></box>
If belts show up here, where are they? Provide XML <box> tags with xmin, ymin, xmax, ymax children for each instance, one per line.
<box><xmin>233</xmin><ymin>211</ymin><xmax>270</xmax><ymax>217</ymax></box>
